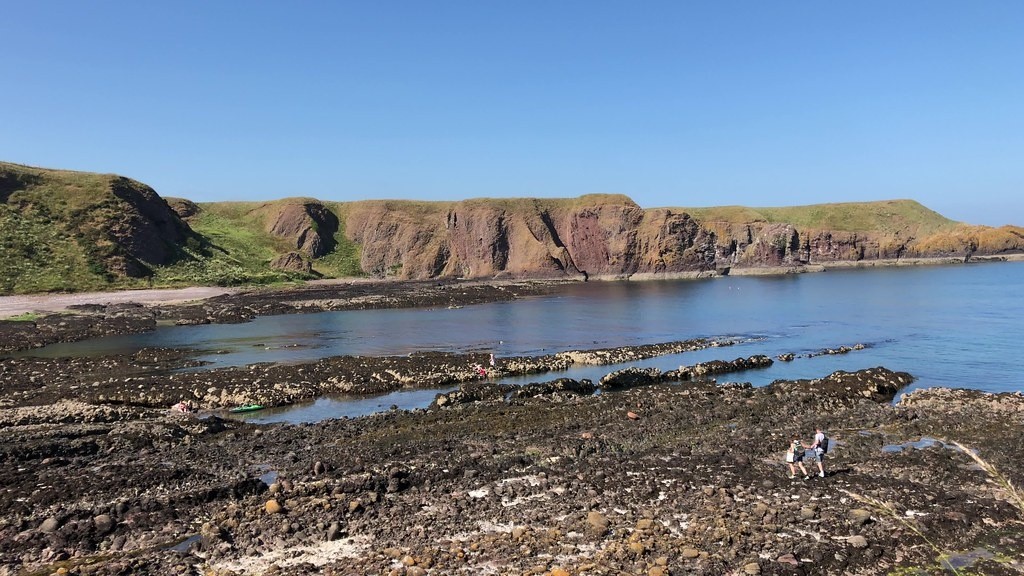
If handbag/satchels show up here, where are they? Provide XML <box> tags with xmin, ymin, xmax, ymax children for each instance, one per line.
<box><xmin>786</xmin><ymin>451</ymin><xmax>794</xmax><ymax>462</ymax></box>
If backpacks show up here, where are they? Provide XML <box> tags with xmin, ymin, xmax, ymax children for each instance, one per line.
<box><xmin>818</xmin><ymin>434</ymin><xmax>828</xmax><ymax>454</ymax></box>
<box><xmin>795</xmin><ymin>441</ymin><xmax>805</xmax><ymax>456</ymax></box>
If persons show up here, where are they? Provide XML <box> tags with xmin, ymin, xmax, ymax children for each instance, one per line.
<box><xmin>810</xmin><ymin>427</ymin><xmax>828</xmax><ymax>477</ymax></box>
<box><xmin>788</xmin><ymin>434</ymin><xmax>811</xmax><ymax>480</ymax></box>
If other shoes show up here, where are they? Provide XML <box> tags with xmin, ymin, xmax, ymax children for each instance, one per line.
<box><xmin>819</xmin><ymin>472</ymin><xmax>824</xmax><ymax>477</ymax></box>
<box><xmin>789</xmin><ymin>475</ymin><xmax>795</xmax><ymax>479</ymax></box>
<box><xmin>804</xmin><ymin>476</ymin><xmax>809</xmax><ymax>481</ymax></box>
